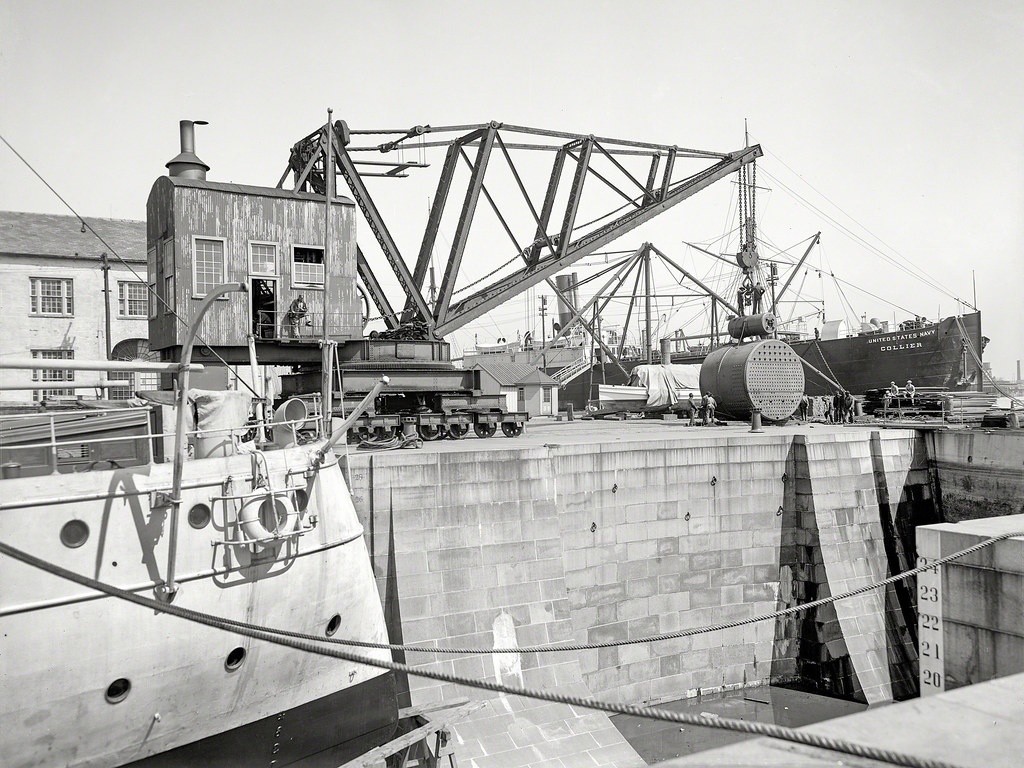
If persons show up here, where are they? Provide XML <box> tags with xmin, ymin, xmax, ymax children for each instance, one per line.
<box><xmin>891</xmin><ymin>381</ymin><xmax>899</xmax><ymax>398</ymax></box>
<box><xmin>285</xmin><ymin>294</ymin><xmax>311</xmax><ymax>343</ymax></box>
<box><xmin>753</xmin><ymin>283</ymin><xmax>764</xmax><ymax>315</ymax></box>
<box><xmin>688</xmin><ymin>393</ymin><xmax>698</xmax><ymax>425</ymax></box>
<box><xmin>843</xmin><ymin>391</ymin><xmax>854</xmax><ymax>424</ymax></box>
<box><xmin>704</xmin><ymin>393</ymin><xmax>717</xmax><ymax>427</ymax></box>
<box><xmin>37</xmin><ymin>401</ymin><xmax>47</xmax><ymax>413</ymax></box>
<box><xmin>253</xmin><ymin>308</ymin><xmax>273</xmax><ymax>339</ymax></box>
<box><xmin>882</xmin><ymin>390</ymin><xmax>892</xmax><ymax>407</ymax></box>
<box><xmin>832</xmin><ymin>390</ymin><xmax>845</xmax><ymax>424</ymax></box>
<box><xmin>701</xmin><ymin>391</ymin><xmax>710</xmax><ymax>426</ymax></box>
<box><xmin>738</xmin><ymin>287</ymin><xmax>749</xmax><ymax>317</ymax></box>
<box><xmin>904</xmin><ymin>380</ymin><xmax>915</xmax><ymax>406</ymax></box>
<box><xmin>799</xmin><ymin>395</ymin><xmax>808</xmax><ymax>421</ymax></box>
<box><xmin>824</xmin><ymin>396</ymin><xmax>835</xmax><ymax>425</ymax></box>
<box><xmin>815</xmin><ymin>328</ymin><xmax>819</xmax><ymax>342</ymax></box>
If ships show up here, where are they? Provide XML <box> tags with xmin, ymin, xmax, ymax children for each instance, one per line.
<box><xmin>462</xmin><ymin>272</ymin><xmax>984</xmax><ymax>412</ymax></box>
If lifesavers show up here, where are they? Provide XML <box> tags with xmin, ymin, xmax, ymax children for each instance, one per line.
<box><xmin>241</xmin><ymin>486</ymin><xmax>298</xmax><ymax>546</ymax></box>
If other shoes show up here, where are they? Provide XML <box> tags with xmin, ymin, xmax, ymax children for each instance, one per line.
<box><xmin>305</xmin><ymin>323</ymin><xmax>313</xmax><ymax>327</ymax></box>
